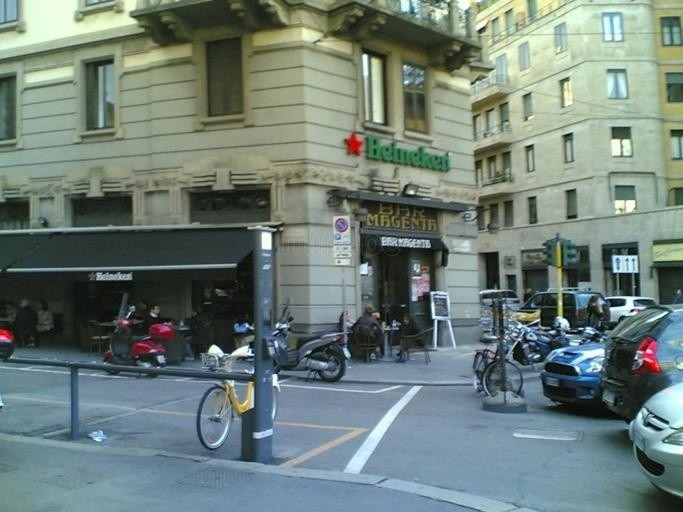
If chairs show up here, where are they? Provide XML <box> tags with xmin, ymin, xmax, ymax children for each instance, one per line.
<box><xmin>356</xmin><ymin>328</ymin><xmax>434</xmax><ymax>363</ymax></box>
<box><xmin>1</xmin><ymin>313</ymin><xmax>214</xmax><ymax>356</ymax></box>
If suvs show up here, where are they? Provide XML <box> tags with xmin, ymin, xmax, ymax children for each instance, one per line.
<box><xmin>602</xmin><ymin>305</ymin><xmax>683</xmax><ymax>424</ymax></box>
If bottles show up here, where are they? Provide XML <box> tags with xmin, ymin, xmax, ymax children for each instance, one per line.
<box><xmin>392</xmin><ymin>319</ymin><xmax>396</xmax><ymax>329</ymax></box>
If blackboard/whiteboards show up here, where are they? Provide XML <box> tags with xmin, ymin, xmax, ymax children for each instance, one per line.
<box><xmin>430</xmin><ymin>291</ymin><xmax>451</xmax><ymax>320</ymax></box>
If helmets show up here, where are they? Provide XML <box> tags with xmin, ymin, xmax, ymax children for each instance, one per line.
<box><xmin>554</xmin><ymin>318</ymin><xmax>570</xmax><ymax>331</ymax></box>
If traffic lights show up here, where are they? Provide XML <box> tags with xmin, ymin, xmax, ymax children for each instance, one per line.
<box><xmin>563</xmin><ymin>240</ymin><xmax>576</xmax><ymax>268</ymax></box>
<box><xmin>541</xmin><ymin>240</ymin><xmax>551</xmax><ymax>264</ymax></box>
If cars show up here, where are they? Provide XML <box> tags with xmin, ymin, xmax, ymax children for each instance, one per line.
<box><xmin>540</xmin><ymin>343</ymin><xmax>603</xmax><ymax>402</ymax></box>
<box><xmin>479</xmin><ymin>288</ymin><xmax>657</xmax><ymax>329</ymax></box>
<box><xmin>628</xmin><ymin>382</ymin><xmax>683</xmax><ymax>497</ymax></box>
<box><xmin>0</xmin><ymin>328</ymin><xmax>15</xmax><ymax>360</ymax></box>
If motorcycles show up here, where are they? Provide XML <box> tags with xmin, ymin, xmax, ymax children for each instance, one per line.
<box><xmin>273</xmin><ymin>300</ymin><xmax>349</xmax><ymax>382</ymax></box>
<box><xmin>481</xmin><ymin>308</ymin><xmax>600</xmax><ymax>369</ymax></box>
<box><xmin>102</xmin><ymin>306</ymin><xmax>173</xmax><ymax>377</ymax></box>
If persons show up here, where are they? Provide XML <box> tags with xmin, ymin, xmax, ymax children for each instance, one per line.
<box><xmin>586</xmin><ymin>294</ymin><xmax>611</xmax><ymax>333</ymax></box>
<box><xmin>671</xmin><ymin>289</ymin><xmax>683</xmax><ymax>303</ymax></box>
<box><xmin>522</xmin><ymin>287</ymin><xmax>534</xmax><ymax>304</ymax></box>
<box><xmin>1</xmin><ymin>298</ymin><xmax>54</xmax><ymax>348</ymax></box>
<box><xmin>339</xmin><ymin>304</ymin><xmax>385</xmax><ymax>359</ymax></box>
<box><xmin>184</xmin><ymin>305</ymin><xmax>209</xmax><ymax>360</ymax></box>
<box><xmin>132</xmin><ymin>298</ymin><xmax>163</xmax><ymax>336</ymax></box>
<box><xmin>396</xmin><ymin>313</ymin><xmax>418</xmax><ymax>356</ymax></box>
<box><xmin>234</xmin><ymin>280</ymin><xmax>252</xmax><ymax>298</ymax></box>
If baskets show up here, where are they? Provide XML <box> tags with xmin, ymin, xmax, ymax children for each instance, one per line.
<box><xmin>200</xmin><ymin>352</ymin><xmax>230</xmax><ymax>373</ymax></box>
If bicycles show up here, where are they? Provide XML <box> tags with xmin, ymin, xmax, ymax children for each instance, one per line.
<box><xmin>196</xmin><ymin>352</ymin><xmax>280</xmax><ymax>449</ymax></box>
<box><xmin>472</xmin><ymin>347</ymin><xmax>522</xmax><ymax>395</ymax></box>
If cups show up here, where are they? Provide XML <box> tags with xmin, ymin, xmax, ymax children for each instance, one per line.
<box><xmin>382</xmin><ymin>321</ymin><xmax>386</xmax><ymax>328</ymax></box>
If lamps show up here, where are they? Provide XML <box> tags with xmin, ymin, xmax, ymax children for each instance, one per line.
<box><xmin>462</xmin><ymin>208</ymin><xmax>500</xmax><ymax>235</ymax></box>
<box><xmin>193</xmin><ymin>190</ymin><xmax>269</xmax><ymax>211</ymax></box>
<box><xmin>327</xmin><ymin>190</ymin><xmax>370</xmax><ymax>223</ymax></box>
<box><xmin>404</xmin><ymin>183</ymin><xmax>421</xmax><ymax>198</ymax></box>
